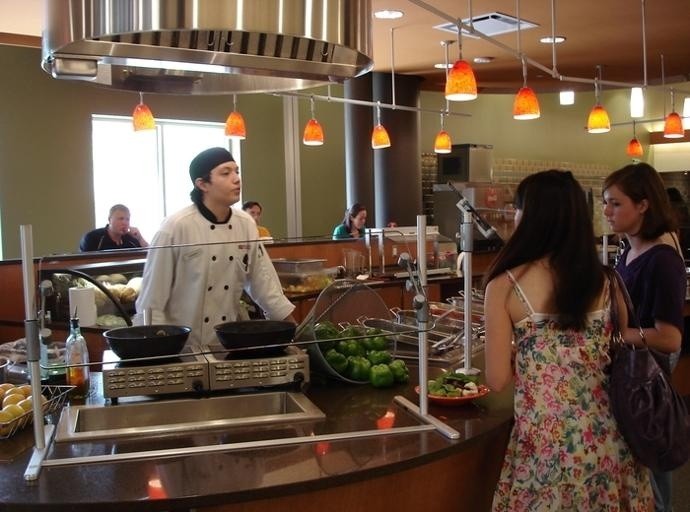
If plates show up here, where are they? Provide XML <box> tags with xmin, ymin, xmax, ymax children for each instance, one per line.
<box><xmin>414</xmin><ymin>382</ymin><xmax>490</xmax><ymax>406</ymax></box>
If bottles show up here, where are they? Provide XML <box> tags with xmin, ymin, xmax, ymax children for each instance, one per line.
<box><xmin>65</xmin><ymin>316</ymin><xmax>91</xmax><ymax>399</ymax></box>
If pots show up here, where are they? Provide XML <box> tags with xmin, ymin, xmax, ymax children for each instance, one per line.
<box><xmin>103</xmin><ymin>321</ymin><xmax>192</xmax><ymax>366</ymax></box>
<box><xmin>213</xmin><ymin>319</ymin><xmax>297</xmax><ymax>361</ymax></box>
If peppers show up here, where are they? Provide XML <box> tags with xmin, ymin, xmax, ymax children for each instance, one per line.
<box><xmin>314</xmin><ymin>319</ymin><xmax>411</xmax><ymax>387</ymax></box>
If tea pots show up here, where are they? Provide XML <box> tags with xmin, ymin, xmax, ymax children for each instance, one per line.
<box><xmin>343</xmin><ymin>248</ymin><xmax>365</xmax><ymax>276</ymax></box>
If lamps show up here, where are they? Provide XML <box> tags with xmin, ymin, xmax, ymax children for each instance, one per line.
<box><xmin>584</xmin><ymin>116</ymin><xmax>690</xmax><ymax>157</ymax></box>
<box><xmin>561</xmin><ymin>4</ymin><xmax>690</xmax><ymax>139</ymax></box>
<box><xmin>414</xmin><ymin>1</ymin><xmax>561</xmax><ymax>123</ymax></box>
<box><xmin>123</xmin><ymin>82</ymin><xmax>470</xmax><ymax>163</ymax></box>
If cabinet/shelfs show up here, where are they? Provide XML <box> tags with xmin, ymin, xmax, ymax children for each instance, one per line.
<box><xmin>331</xmin><ymin>288</ymin><xmax>403</xmax><ymax>321</ymax></box>
<box><xmin>404</xmin><ymin>280</ymin><xmax>474</xmax><ymax>311</ymax></box>
<box><xmin>42</xmin><ymin>256</ymin><xmax>149</xmax><ymax>330</ymax></box>
<box><xmin>288</xmin><ymin>297</ymin><xmax>332</xmax><ymax>324</ymax></box>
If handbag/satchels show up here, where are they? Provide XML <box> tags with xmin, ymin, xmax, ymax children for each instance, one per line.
<box><xmin>605</xmin><ymin>263</ymin><xmax>690</xmax><ymax>472</ymax></box>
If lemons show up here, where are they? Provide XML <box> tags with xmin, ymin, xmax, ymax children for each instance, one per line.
<box><xmin>1</xmin><ymin>382</ymin><xmax>47</xmax><ymax>423</ymax></box>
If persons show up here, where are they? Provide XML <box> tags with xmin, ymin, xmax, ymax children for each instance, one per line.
<box><xmin>131</xmin><ymin>148</ymin><xmax>300</xmax><ymax>326</ymax></box>
<box><xmin>601</xmin><ymin>162</ymin><xmax>689</xmax><ymax>512</ymax></box>
<box><xmin>386</xmin><ymin>217</ymin><xmax>399</xmax><ymax>228</ymax></box>
<box><xmin>240</xmin><ymin>202</ymin><xmax>270</xmax><ymax>238</ymax></box>
<box><xmin>331</xmin><ymin>202</ymin><xmax>366</xmax><ymax>240</ymax></box>
<box><xmin>80</xmin><ymin>204</ymin><xmax>149</xmax><ymax>252</ymax></box>
<box><xmin>481</xmin><ymin>170</ymin><xmax>656</xmax><ymax>512</ymax></box>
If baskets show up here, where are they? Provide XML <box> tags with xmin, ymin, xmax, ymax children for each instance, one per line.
<box><xmin>0</xmin><ymin>377</ymin><xmax>77</xmax><ymax>441</ymax></box>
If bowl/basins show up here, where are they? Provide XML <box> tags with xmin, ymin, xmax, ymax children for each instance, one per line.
<box><xmin>1</xmin><ymin>355</ymin><xmax>9</xmax><ymax>383</ymax></box>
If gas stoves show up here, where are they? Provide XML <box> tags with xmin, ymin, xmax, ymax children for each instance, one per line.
<box><xmin>101</xmin><ymin>343</ymin><xmax>310</xmax><ymax>402</ymax></box>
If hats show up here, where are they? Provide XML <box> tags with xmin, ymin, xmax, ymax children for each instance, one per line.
<box><xmin>188</xmin><ymin>147</ymin><xmax>235</xmax><ymax>182</ymax></box>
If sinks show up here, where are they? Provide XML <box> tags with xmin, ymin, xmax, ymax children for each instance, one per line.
<box><xmin>53</xmin><ymin>391</ymin><xmax>329</xmax><ymax>444</ymax></box>
